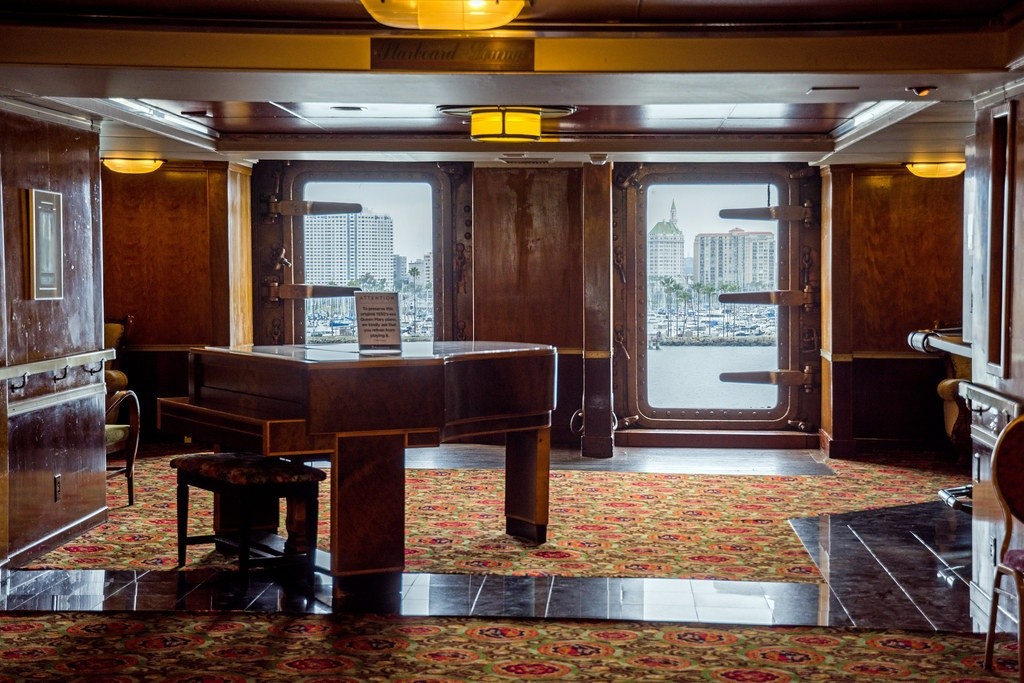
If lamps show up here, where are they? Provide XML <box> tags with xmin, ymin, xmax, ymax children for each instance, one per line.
<box><xmin>436</xmin><ymin>105</ymin><xmax>578</xmax><ymax>142</ymax></box>
<box><xmin>905</xmin><ymin>86</ymin><xmax>938</xmax><ymax>97</ymax></box>
<box><xmin>99</xmin><ymin>158</ymin><xmax>168</xmax><ymax>175</ymax></box>
<box><xmin>900</xmin><ymin>162</ymin><xmax>966</xmax><ymax>179</ymax></box>
<box><xmin>356</xmin><ymin>0</ymin><xmax>534</xmax><ymax>31</ymax></box>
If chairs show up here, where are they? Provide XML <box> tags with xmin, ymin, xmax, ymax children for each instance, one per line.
<box><xmin>106</xmin><ymin>389</ymin><xmax>141</xmax><ymax>505</ymax></box>
<box><xmin>985</xmin><ymin>415</ymin><xmax>1024</xmax><ymax>683</ymax></box>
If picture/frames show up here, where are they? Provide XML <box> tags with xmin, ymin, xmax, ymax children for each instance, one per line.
<box><xmin>27</xmin><ymin>188</ymin><xmax>64</xmax><ymax>300</ymax></box>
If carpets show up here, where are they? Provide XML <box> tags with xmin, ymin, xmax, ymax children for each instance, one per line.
<box><xmin>19</xmin><ymin>455</ymin><xmax>971</xmax><ymax>585</ymax></box>
<box><xmin>0</xmin><ymin>606</ymin><xmax>1024</xmax><ymax>683</ymax></box>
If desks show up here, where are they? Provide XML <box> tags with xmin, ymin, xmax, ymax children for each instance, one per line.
<box><xmin>155</xmin><ymin>338</ymin><xmax>559</xmax><ymax>577</ymax></box>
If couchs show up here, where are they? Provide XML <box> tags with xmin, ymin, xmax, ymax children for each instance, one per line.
<box><xmin>931</xmin><ymin>327</ymin><xmax>973</xmax><ymax>467</ymax></box>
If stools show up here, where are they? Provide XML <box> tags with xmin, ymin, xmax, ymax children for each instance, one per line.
<box><xmin>170</xmin><ymin>451</ymin><xmax>328</xmax><ymax>578</ymax></box>
